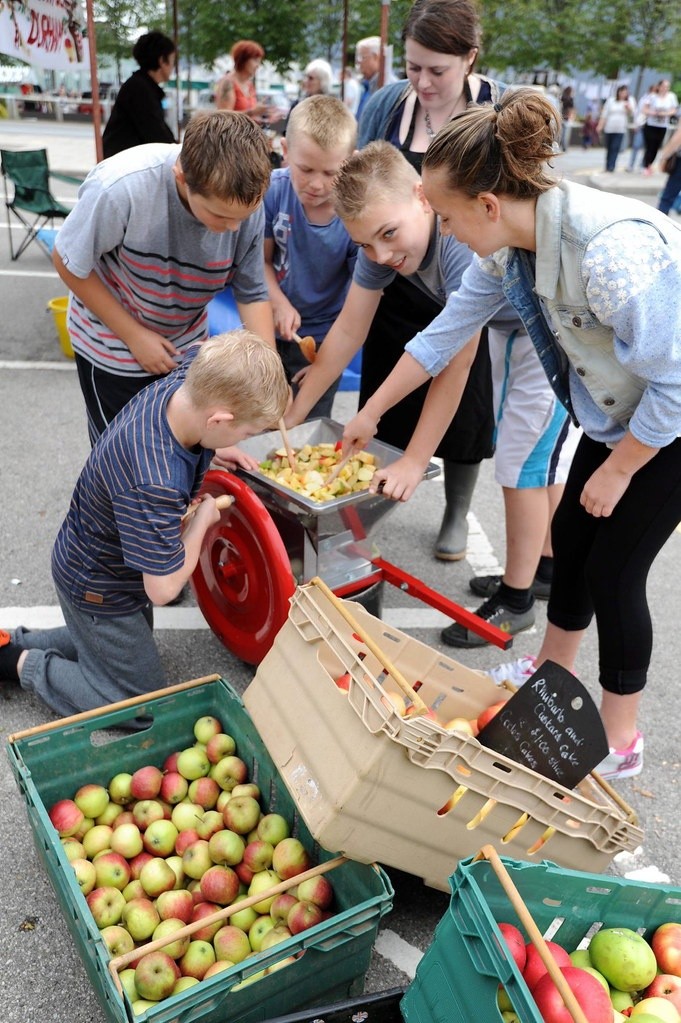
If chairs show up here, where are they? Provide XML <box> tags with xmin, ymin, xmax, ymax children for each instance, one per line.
<box><xmin>0</xmin><ymin>149</ymin><xmax>71</xmax><ymax>260</ymax></box>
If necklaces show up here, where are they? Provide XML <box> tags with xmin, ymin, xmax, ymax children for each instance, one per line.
<box><xmin>425</xmin><ymin>91</ymin><xmax>464</xmax><ymax>139</ymax></box>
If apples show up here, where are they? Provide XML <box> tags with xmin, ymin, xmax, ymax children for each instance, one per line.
<box><xmin>52</xmin><ymin>718</ymin><xmax>330</xmax><ymax>1019</ymax></box>
<box><xmin>255</xmin><ymin>443</ymin><xmax>383</xmax><ymax>505</ymax></box>
<box><xmin>336</xmin><ymin>671</ymin><xmax>574</xmax><ymax>854</ymax></box>
<box><xmin>494</xmin><ymin>922</ymin><xmax>681</xmax><ymax>1023</ymax></box>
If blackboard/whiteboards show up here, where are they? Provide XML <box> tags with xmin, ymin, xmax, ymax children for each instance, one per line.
<box><xmin>475</xmin><ymin>659</ymin><xmax>610</xmax><ymax>798</ymax></box>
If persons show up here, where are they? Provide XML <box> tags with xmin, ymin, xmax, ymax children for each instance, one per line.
<box><xmin>546</xmin><ymin>83</ymin><xmax>574</xmax><ymax>153</ymax></box>
<box><xmin>0</xmin><ymin>330</ymin><xmax>287</xmax><ymax>734</ymax></box>
<box><xmin>354</xmin><ymin>36</ymin><xmax>401</xmax><ymax>122</ymax></box>
<box><xmin>282</xmin><ymin>141</ymin><xmax>569</xmax><ymax>648</ymax></box>
<box><xmin>261</xmin><ymin>92</ymin><xmax>360</xmax><ymax>422</ymax></box>
<box><xmin>420</xmin><ymin>85</ymin><xmax>681</xmax><ymax>779</ymax></box>
<box><xmin>50</xmin><ymin>109</ymin><xmax>292</xmax><ymax>454</ymax></box>
<box><xmin>283</xmin><ymin>57</ymin><xmax>337</xmax><ymax>135</ymax></box>
<box><xmin>217</xmin><ymin>39</ymin><xmax>283</xmax><ymax>129</ymax></box>
<box><xmin>357</xmin><ymin>0</ymin><xmax>510</xmax><ymax>560</ymax></box>
<box><xmin>101</xmin><ymin>31</ymin><xmax>181</xmax><ymax>163</ymax></box>
<box><xmin>21</xmin><ymin>82</ymin><xmax>81</xmax><ymax>117</ymax></box>
<box><xmin>583</xmin><ymin>79</ymin><xmax>681</xmax><ymax>219</ymax></box>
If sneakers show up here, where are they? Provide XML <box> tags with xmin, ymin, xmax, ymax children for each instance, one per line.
<box><xmin>468</xmin><ymin>573</ymin><xmax>552</xmax><ymax>601</ymax></box>
<box><xmin>441</xmin><ymin>593</ymin><xmax>535</xmax><ymax>649</ymax></box>
<box><xmin>590</xmin><ymin>731</ymin><xmax>644</xmax><ymax>782</ymax></box>
<box><xmin>484</xmin><ymin>657</ymin><xmax>576</xmax><ymax>689</ymax></box>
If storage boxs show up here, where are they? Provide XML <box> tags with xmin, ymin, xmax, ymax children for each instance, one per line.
<box><xmin>400</xmin><ymin>844</ymin><xmax>681</xmax><ymax>1023</ymax></box>
<box><xmin>242</xmin><ymin>576</ymin><xmax>645</xmax><ymax>896</ymax></box>
<box><xmin>7</xmin><ymin>672</ymin><xmax>394</xmax><ymax>1023</ymax></box>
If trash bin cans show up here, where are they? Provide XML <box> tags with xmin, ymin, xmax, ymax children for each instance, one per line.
<box><xmin>46</xmin><ymin>296</ymin><xmax>75</xmax><ymax>358</ymax></box>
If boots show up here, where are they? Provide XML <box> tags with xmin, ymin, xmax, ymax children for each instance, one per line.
<box><xmin>434</xmin><ymin>458</ymin><xmax>480</xmax><ymax>560</ymax></box>
